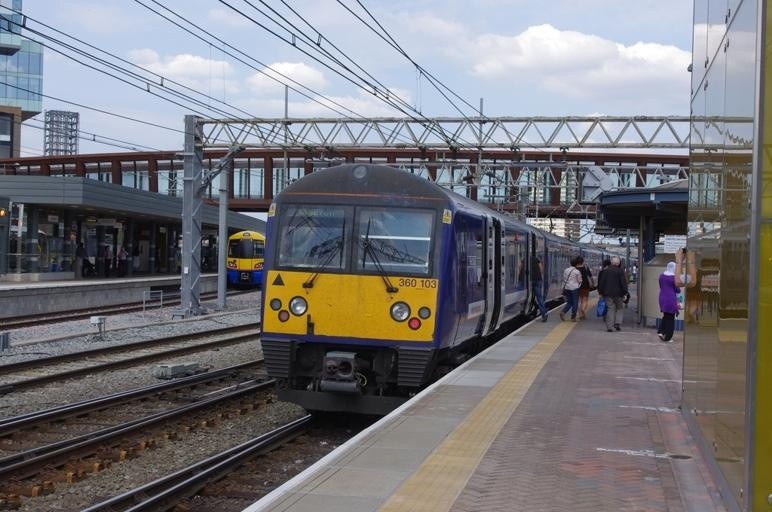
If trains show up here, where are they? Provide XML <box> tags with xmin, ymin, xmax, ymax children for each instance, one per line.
<box><xmin>227</xmin><ymin>230</ymin><xmax>266</xmax><ymax>289</ymax></box>
<box><xmin>258</xmin><ymin>163</ymin><xmax>644</xmax><ymax>417</ymax></box>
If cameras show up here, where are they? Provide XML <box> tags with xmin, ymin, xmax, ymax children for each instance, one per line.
<box><xmin>680</xmin><ymin>248</ymin><xmax>686</xmax><ymax>253</ymax></box>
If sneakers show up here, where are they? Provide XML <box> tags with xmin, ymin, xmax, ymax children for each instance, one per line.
<box><xmin>539</xmin><ymin>309</ymin><xmax>587</xmax><ymax>322</ymax></box>
<box><xmin>606</xmin><ymin>324</ymin><xmax>623</xmax><ymax>332</ymax></box>
<box><xmin>658</xmin><ymin>332</ymin><xmax>674</xmax><ymax>343</ymax></box>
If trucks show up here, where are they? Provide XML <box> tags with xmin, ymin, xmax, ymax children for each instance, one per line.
<box><xmin>172</xmin><ymin>229</ymin><xmax>218</xmax><ymax>274</ymax></box>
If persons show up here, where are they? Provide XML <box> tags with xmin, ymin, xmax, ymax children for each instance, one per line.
<box><xmin>117</xmin><ymin>246</ymin><xmax>130</xmax><ymax>277</ymax></box>
<box><xmin>558</xmin><ymin>254</ymin><xmax>640</xmax><ymax>332</ymax></box>
<box><xmin>76</xmin><ymin>242</ymin><xmax>90</xmax><ymax>281</ymax></box>
<box><xmin>674</xmin><ymin>246</ymin><xmax>686</xmax><ymax>287</ymax></box>
<box><xmin>684</xmin><ymin>248</ymin><xmax>699</xmax><ymax>289</ymax></box>
<box><xmin>532</xmin><ymin>255</ymin><xmax>548</xmax><ymax>323</ymax></box>
<box><xmin>656</xmin><ymin>261</ymin><xmax>681</xmax><ymax>343</ymax></box>
<box><xmin>104</xmin><ymin>244</ymin><xmax>113</xmax><ymax>277</ymax></box>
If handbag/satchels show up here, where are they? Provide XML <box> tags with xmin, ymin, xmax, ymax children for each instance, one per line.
<box><xmin>561</xmin><ymin>287</ymin><xmax>567</xmax><ymax>294</ymax></box>
<box><xmin>596</xmin><ymin>296</ymin><xmax>607</xmax><ymax>316</ymax></box>
